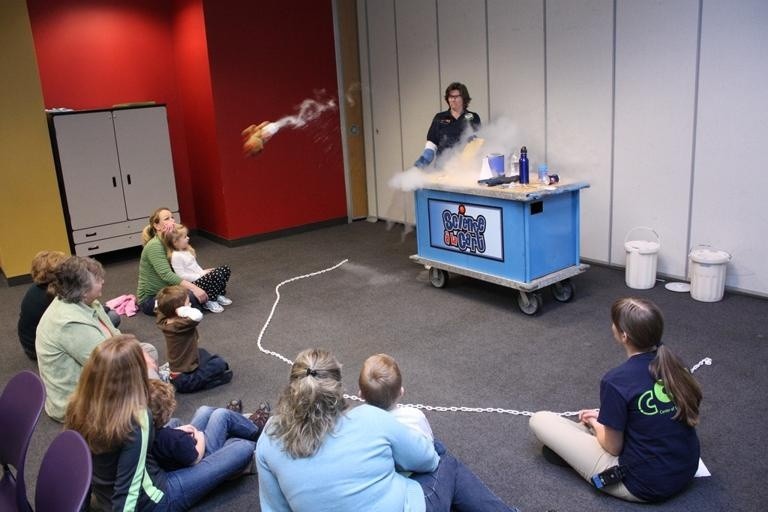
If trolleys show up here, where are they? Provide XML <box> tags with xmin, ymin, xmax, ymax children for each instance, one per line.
<box><xmin>406</xmin><ymin>166</ymin><xmax>592</xmax><ymax>314</ymax></box>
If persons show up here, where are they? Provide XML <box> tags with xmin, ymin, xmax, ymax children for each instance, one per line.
<box><xmin>526</xmin><ymin>296</ymin><xmax>703</xmax><ymax>506</ymax></box>
<box><xmin>414</xmin><ymin>81</ymin><xmax>482</xmax><ymax>173</ymax></box>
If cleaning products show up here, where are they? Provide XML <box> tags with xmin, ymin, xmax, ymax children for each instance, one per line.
<box><xmin>519</xmin><ymin>146</ymin><xmax>530</xmax><ymax>185</ymax></box>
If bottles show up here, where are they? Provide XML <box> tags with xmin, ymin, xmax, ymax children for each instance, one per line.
<box><xmin>538</xmin><ymin>162</ymin><xmax>548</xmax><ymax>179</ymax></box>
<box><xmin>478</xmin><ymin>145</ymin><xmax>531</xmax><ymax>185</ymax></box>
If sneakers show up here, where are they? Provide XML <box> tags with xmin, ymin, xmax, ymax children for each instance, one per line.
<box><xmin>201</xmin><ymin>294</ymin><xmax>233</xmax><ymax>314</ymax></box>
<box><xmin>540</xmin><ymin>444</ymin><xmax>569</xmax><ymax>468</ymax></box>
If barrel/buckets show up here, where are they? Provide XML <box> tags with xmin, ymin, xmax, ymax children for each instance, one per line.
<box><xmin>624</xmin><ymin>226</ymin><xmax>660</xmax><ymax>290</ymax></box>
<box><xmin>689</xmin><ymin>243</ymin><xmax>731</xmax><ymax>304</ymax></box>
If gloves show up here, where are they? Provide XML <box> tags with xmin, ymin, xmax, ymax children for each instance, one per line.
<box><xmin>414</xmin><ymin>147</ymin><xmax>435</xmax><ymax>170</ymax></box>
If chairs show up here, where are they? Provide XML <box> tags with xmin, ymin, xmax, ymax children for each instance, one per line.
<box><xmin>34</xmin><ymin>429</ymin><xmax>91</xmax><ymax>510</ymax></box>
<box><xmin>1</xmin><ymin>369</ymin><xmax>47</xmax><ymax>512</ymax></box>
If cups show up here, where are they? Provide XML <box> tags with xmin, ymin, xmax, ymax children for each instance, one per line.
<box><xmin>542</xmin><ymin>173</ymin><xmax>559</xmax><ymax>186</ymax></box>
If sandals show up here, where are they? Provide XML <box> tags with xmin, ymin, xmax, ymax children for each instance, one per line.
<box><xmin>226</xmin><ymin>397</ymin><xmax>242</xmax><ymax>412</ymax></box>
<box><xmin>248</xmin><ymin>398</ymin><xmax>271</xmax><ymax>430</ymax></box>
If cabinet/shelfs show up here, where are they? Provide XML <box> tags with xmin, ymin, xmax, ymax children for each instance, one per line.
<box><xmin>47</xmin><ymin>102</ymin><xmax>183</xmax><ymax>261</ymax></box>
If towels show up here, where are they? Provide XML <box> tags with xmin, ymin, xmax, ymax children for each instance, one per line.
<box><xmin>104</xmin><ymin>292</ymin><xmax>140</xmax><ymax>318</ymax></box>
